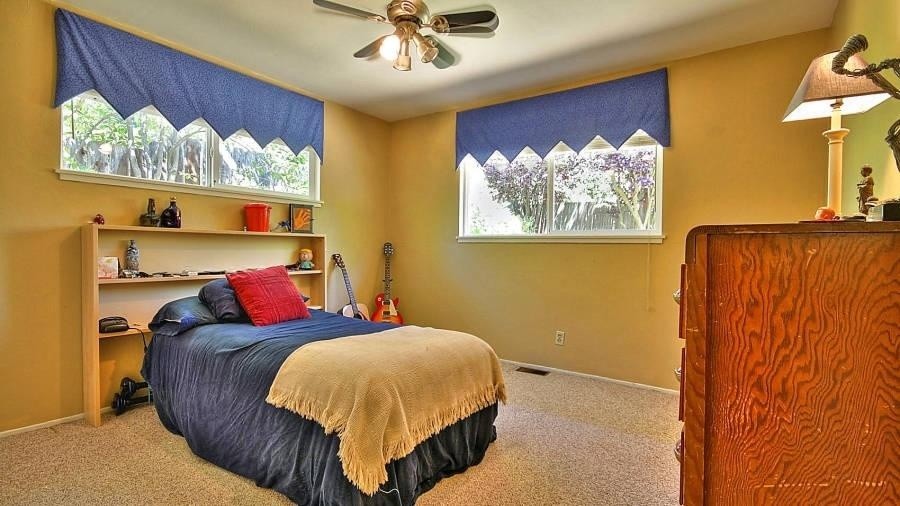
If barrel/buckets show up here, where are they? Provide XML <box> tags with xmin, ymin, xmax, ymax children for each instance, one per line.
<box><xmin>241</xmin><ymin>203</ymin><xmax>271</xmax><ymax>231</ymax></box>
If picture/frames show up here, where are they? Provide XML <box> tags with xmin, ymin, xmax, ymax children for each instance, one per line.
<box><xmin>288</xmin><ymin>203</ymin><xmax>315</xmax><ymax>233</ymax></box>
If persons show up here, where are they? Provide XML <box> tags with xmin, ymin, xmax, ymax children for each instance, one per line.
<box><xmin>856</xmin><ymin>166</ymin><xmax>874</xmax><ymax>215</ymax></box>
<box><xmin>293</xmin><ymin>209</ymin><xmax>310</xmax><ymax>230</ymax></box>
<box><xmin>299</xmin><ymin>248</ymin><xmax>315</xmax><ymax>270</ymax></box>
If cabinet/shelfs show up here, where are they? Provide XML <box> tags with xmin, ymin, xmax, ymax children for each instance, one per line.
<box><xmin>79</xmin><ymin>222</ymin><xmax>329</xmax><ymax>428</ymax></box>
<box><xmin>672</xmin><ymin>221</ymin><xmax>900</xmax><ymax>506</ymax></box>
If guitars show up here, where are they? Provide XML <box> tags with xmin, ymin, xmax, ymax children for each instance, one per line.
<box><xmin>333</xmin><ymin>254</ymin><xmax>370</xmax><ymax>322</ymax></box>
<box><xmin>370</xmin><ymin>243</ymin><xmax>404</xmax><ymax>325</ymax></box>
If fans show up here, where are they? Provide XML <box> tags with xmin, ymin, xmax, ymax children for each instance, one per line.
<box><xmin>312</xmin><ymin>0</ymin><xmax>499</xmax><ymax>69</ymax></box>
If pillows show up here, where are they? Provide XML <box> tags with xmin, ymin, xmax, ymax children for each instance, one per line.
<box><xmin>148</xmin><ymin>265</ymin><xmax>312</xmax><ymax>337</ymax></box>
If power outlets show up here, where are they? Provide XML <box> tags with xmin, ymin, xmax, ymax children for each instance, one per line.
<box><xmin>555</xmin><ymin>331</ymin><xmax>565</xmax><ymax>345</ymax></box>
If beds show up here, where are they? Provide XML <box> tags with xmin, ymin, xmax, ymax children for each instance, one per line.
<box><xmin>140</xmin><ymin>307</ymin><xmax>508</xmax><ymax>506</ymax></box>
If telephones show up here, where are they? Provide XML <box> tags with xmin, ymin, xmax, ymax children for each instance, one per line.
<box><xmin>99</xmin><ymin>316</ymin><xmax>128</xmax><ymax>333</ymax></box>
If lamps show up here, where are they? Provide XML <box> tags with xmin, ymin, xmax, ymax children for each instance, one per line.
<box><xmin>779</xmin><ymin>49</ymin><xmax>900</xmax><ymax>222</ymax></box>
<box><xmin>378</xmin><ymin>21</ymin><xmax>439</xmax><ymax>71</ymax></box>
